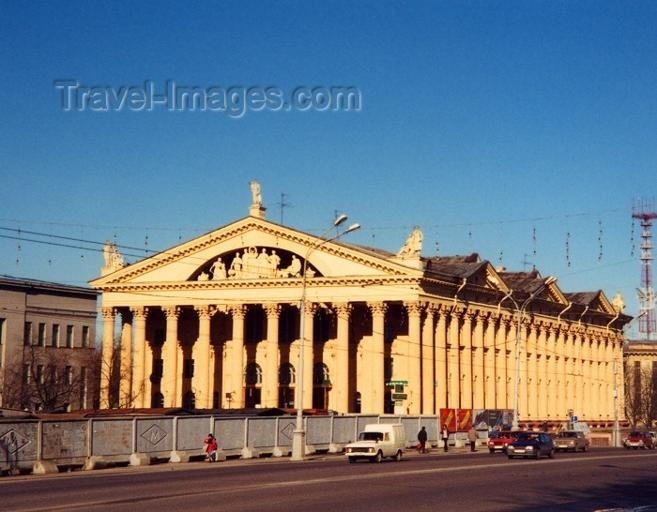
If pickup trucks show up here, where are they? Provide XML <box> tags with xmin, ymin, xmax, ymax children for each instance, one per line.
<box><xmin>344</xmin><ymin>423</ymin><xmax>408</xmax><ymax>467</ymax></box>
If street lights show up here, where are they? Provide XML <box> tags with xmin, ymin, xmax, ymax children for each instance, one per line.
<box><xmin>287</xmin><ymin>212</ymin><xmax>363</xmax><ymax>463</ymax></box>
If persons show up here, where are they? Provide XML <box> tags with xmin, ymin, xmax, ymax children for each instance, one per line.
<box><xmin>208</xmin><ymin>244</ymin><xmax>302</xmax><ymax>280</ymax></box>
<box><xmin>439</xmin><ymin>424</ymin><xmax>451</xmax><ymax>452</ymax></box>
<box><xmin>418</xmin><ymin>426</ymin><xmax>427</xmax><ymax>454</ymax></box>
<box><xmin>204</xmin><ymin>433</ymin><xmax>217</xmax><ymax>462</ymax></box>
<box><xmin>468</xmin><ymin>425</ymin><xmax>479</xmax><ymax>452</ymax></box>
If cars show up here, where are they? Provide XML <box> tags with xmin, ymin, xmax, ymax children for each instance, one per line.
<box><xmin>487</xmin><ymin>429</ymin><xmax>590</xmax><ymax>460</ymax></box>
<box><xmin>621</xmin><ymin>430</ymin><xmax>657</xmax><ymax>450</ymax></box>
